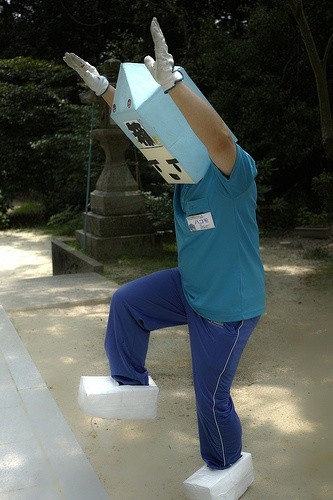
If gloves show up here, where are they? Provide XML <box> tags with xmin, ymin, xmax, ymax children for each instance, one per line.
<box><xmin>63</xmin><ymin>52</ymin><xmax>109</xmax><ymax>96</ymax></box>
<box><xmin>144</xmin><ymin>17</ymin><xmax>184</xmax><ymax>94</ymax></box>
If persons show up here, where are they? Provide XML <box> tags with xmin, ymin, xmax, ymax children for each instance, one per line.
<box><xmin>63</xmin><ymin>17</ymin><xmax>266</xmax><ymax>500</ymax></box>
